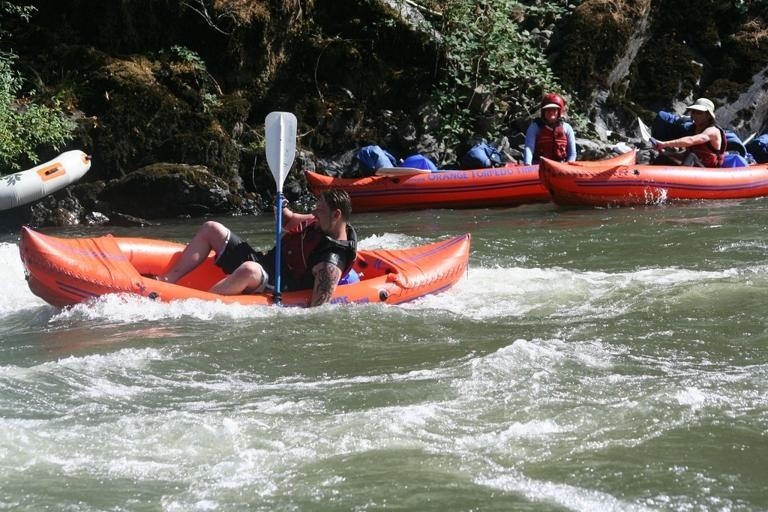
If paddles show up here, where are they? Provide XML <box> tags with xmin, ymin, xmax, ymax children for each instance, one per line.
<box><xmin>638</xmin><ymin>116</ymin><xmax>663</xmax><ymax>152</ymax></box>
<box><xmin>264</xmin><ymin>111</ymin><xmax>298</xmax><ymax>303</ymax></box>
<box><xmin>375</xmin><ymin>166</ymin><xmax>456</xmax><ymax>176</ymax></box>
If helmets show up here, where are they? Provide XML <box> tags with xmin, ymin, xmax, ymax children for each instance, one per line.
<box><xmin>541</xmin><ymin>91</ymin><xmax>566</xmax><ymax>122</ymax></box>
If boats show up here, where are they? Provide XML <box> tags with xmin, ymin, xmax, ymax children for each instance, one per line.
<box><xmin>0</xmin><ymin>148</ymin><xmax>92</xmax><ymax>210</ymax></box>
<box><xmin>304</xmin><ymin>148</ymin><xmax>642</xmax><ymax>214</ymax></box>
<box><xmin>535</xmin><ymin>155</ymin><xmax>768</xmax><ymax>203</ymax></box>
<box><xmin>16</xmin><ymin>223</ymin><xmax>475</xmax><ymax>315</ymax></box>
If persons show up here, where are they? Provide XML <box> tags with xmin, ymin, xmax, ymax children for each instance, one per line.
<box><xmin>152</xmin><ymin>189</ymin><xmax>358</xmax><ymax>309</ymax></box>
<box><xmin>523</xmin><ymin>93</ymin><xmax>577</xmax><ymax>166</ymax></box>
<box><xmin>652</xmin><ymin>98</ymin><xmax>728</xmax><ymax>169</ymax></box>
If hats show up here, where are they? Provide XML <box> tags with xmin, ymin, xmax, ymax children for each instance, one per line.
<box><xmin>689</xmin><ymin>94</ymin><xmax>719</xmax><ymax>121</ymax></box>
